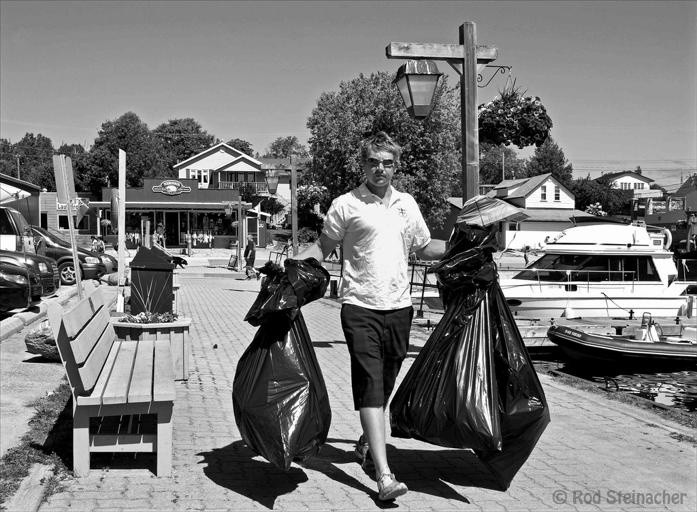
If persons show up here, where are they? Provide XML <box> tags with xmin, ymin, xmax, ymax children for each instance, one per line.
<box><xmin>90</xmin><ymin>235</ymin><xmax>97</xmax><ymax>253</ymax></box>
<box><xmin>124</xmin><ymin>229</ymin><xmax>214</xmax><ymax>249</ymax></box>
<box><xmin>271</xmin><ymin>128</ymin><xmax>453</xmax><ymax>503</ymax></box>
<box><xmin>242</xmin><ymin>234</ymin><xmax>261</xmax><ymax>281</ymax></box>
<box><xmin>95</xmin><ymin>236</ymin><xmax>106</xmax><ymax>254</ymax></box>
<box><xmin>328</xmin><ymin>246</ymin><xmax>339</xmax><ymax>260</ymax></box>
<box><xmin>522</xmin><ymin>244</ymin><xmax>532</xmax><ymax>268</ymax></box>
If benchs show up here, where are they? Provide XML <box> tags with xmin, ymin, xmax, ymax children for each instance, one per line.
<box><xmin>47</xmin><ymin>280</ymin><xmax>175</xmax><ymax>482</ymax></box>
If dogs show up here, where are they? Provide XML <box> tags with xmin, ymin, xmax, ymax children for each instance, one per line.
<box><xmin>173</xmin><ymin>256</ymin><xmax>188</xmax><ymax>269</ymax></box>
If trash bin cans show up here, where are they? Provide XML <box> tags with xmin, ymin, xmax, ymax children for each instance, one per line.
<box><xmin>130</xmin><ymin>242</ymin><xmax>174</xmax><ymax>315</ymax></box>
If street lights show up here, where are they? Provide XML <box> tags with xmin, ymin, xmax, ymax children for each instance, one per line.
<box><xmin>222</xmin><ymin>196</ymin><xmax>244</xmax><ymax>270</ymax></box>
<box><xmin>386</xmin><ymin>22</ymin><xmax>499</xmax><ymax>214</ymax></box>
<box><xmin>260</xmin><ymin>153</ymin><xmax>299</xmax><ymax>256</ymax></box>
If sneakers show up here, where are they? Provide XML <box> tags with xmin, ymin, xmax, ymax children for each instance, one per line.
<box><xmin>245</xmin><ymin>272</ymin><xmax>262</xmax><ymax>281</ymax></box>
<box><xmin>376</xmin><ymin>473</ymin><xmax>408</xmax><ymax>500</ymax></box>
<box><xmin>353</xmin><ymin>434</ymin><xmax>376</xmax><ymax>478</ymax></box>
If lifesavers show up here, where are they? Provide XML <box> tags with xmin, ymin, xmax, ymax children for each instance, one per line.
<box><xmin>660</xmin><ymin>229</ymin><xmax>672</xmax><ymax>249</ymax></box>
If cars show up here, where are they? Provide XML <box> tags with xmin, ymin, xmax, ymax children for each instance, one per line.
<box><xmin>1</xmin><ymin>206</ymin><xmax>117</xmax><ymax>313</ymax></box>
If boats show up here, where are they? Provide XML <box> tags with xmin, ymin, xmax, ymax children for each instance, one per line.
<box><xmin>426</xmin><ymin>215</ymin><xmax>696</xmax><ymax>346</ymax></box>
<box><xmin>548</xmin><ymin>312</ymin><xmax>697</xmax><ymax>374</ymax></box>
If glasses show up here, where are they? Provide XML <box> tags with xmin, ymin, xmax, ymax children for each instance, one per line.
<box><xmin>365</xmin><ymin>158</ymin><xmax>395</xmax><ymax>167</ymax></box>
